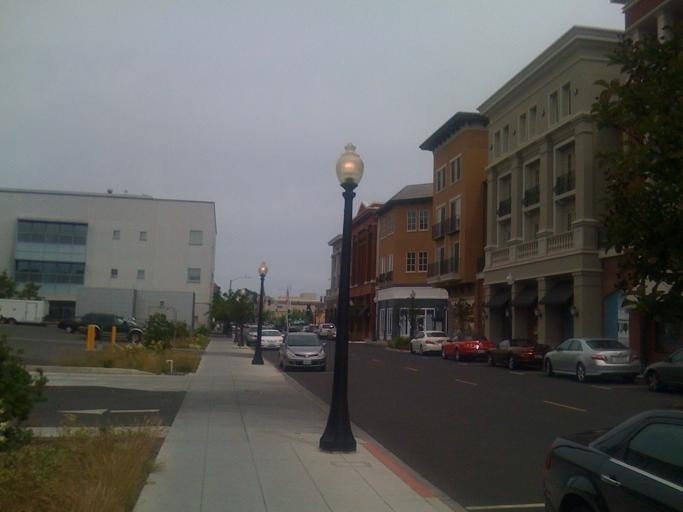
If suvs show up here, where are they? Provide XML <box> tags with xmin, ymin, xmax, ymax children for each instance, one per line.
<box><xmin>78</xmin><ymin>312</ymin><xmax>148</xmax><ymax>344</ymax></box>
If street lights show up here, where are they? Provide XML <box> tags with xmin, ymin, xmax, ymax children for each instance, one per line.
<box><xmin>316</xmin><ymin>142</ymin><xmax>366</xmax><ymax>456</ymax></box>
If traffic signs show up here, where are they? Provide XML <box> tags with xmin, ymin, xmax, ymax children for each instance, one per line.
<box><xmin>250</xmin><ymin>261</ymin><xmax>269</xmax><ymax>365</ymax></box>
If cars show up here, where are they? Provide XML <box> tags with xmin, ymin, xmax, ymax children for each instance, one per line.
<box><xmin>539</xmin><ymin>402</ymin><xmax>682</xmax><ymax>512</ymax></box>
<box><xmin>408</xmin><ymin>329</ymin><xmax>682</xmax><ymax>394</ymax></box>
<box><xmin>278</xmin><ymin>332</ymin><xmax>327</xmax><ymax>373</ymax></box>
